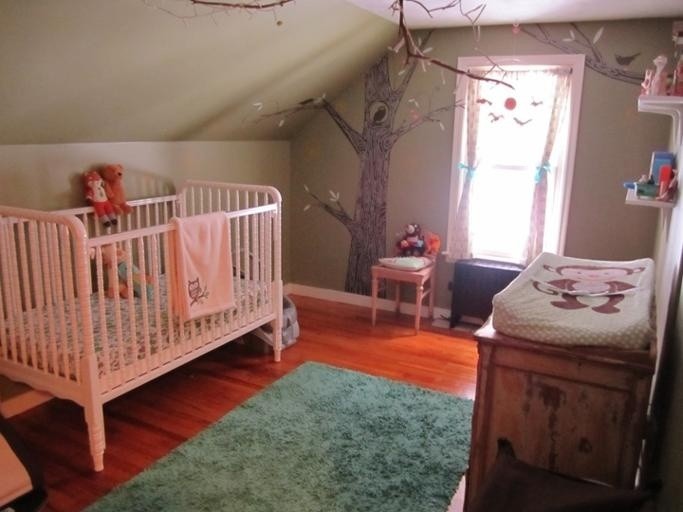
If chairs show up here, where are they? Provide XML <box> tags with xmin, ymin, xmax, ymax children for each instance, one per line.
<box><xmin>371</xmin><ymin>228</ymin><xmax>441</xmax><ymax>336</ymax></box>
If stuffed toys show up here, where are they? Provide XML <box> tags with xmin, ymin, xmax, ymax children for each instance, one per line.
<box><xmin>423</xmin><ymin>230</ymin><xmax>441</xmax><ymax>256</ymax></box>
<box><xmin>116</xmin><ymin>259</ymin><xmax>152</xmax><ymax>302</ymax></box>
<box><xmin>81</xmin><ymin>170</ymin><xmax>119</xmax><ymax>227</ymax></box>
<box><xmin>395</xmin><ymin>222</ymin><xmax>421</xmax><ymax>257</ymax></box>
<box><xmin>99</xmin><ymin>246</ymin><xmax>129</xmax><ymax>299</ymax></box>
<box><xmin>101</xmin><ymin>162</ymin><xmax>134</xmax><ymax>216</ymax></box>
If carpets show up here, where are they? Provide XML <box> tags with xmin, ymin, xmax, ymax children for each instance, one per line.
<box><xmin>78</xmin><ymin>358</ymin><xmax>476</xmax><ymax>511</ymax></box>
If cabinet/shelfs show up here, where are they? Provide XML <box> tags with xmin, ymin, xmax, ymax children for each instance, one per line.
<box><xmin>624</xmin><ymin>96</ymin><xmax>682</xmax><ymax>230</ymax></box>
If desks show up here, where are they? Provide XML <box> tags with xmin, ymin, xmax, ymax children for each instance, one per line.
<box><xmin>449</xmin><ymin>257</ymin><xmax>528</xmax><ymax>328</ymax></box>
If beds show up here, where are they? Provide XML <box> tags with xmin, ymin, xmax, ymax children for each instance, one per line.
<box><xmin>0</xmin><ymin>177</ymin><xmax>284</xmax><ymax>473</ymax></box>
